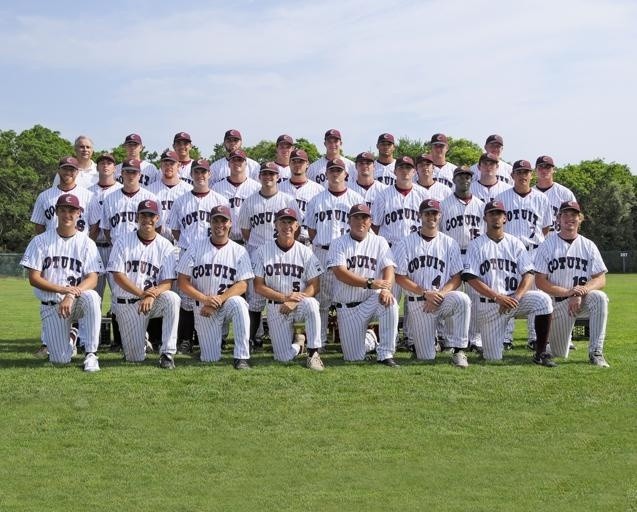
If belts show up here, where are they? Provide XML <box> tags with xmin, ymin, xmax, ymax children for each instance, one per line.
<box><xmin>479</xmin><ymin>297</ymin><xmax>497</xmax><ymax>303</ymax></box>
<box><xmin>41</xmin><ymin>301</ymin><xmax>59</xmax><ymax>306</ymax></box>
<box><xmin>336</xmin><ymin>301</ymin><xmax>362</xmax><ymax>308</ymax></box>
<box><xmin>268</xmin><ymin>299</ymin><xmax>285</xmax><ymax>305</ymax></box>
<box><xmin>551</xmin><ymin>296</ymin><xmax>570</xmax><ymax>302</ymax></box>
<box><xmin>117</xmin><ymin>297</ymin><xmax>141</xmax><ymax>304</ymax></box>
<box><xmin>408</xmin><ymin>296</ymin><xmax>428</xmax><ymax>302</ymax></box>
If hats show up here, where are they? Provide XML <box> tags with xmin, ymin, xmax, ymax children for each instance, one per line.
<box><xmin>276</xmin><ymin>208</ymin><xmax>297</xmax><ymax>221</ymax></box>
<box><xmin>512</xmin><ymin>160</ymin><xmax>532</xmax><ymax>171</ymax></box>
<box><xmin>59</xmin><ymin>156</ymin><xmax>80</xmax><ymax>171</ymax></box>
<box><xmin>453</xmin><ymin>165</ymin><xmax>475</xmax><ymax>177</ymax></box>
<box><xmin>138</xmin><ymin>200</ymin><xmax>159</xmax><ymax>215</ymax></box>
<box><xmin>559</xmin><ymin>200</ymin><xmax>581</xmax><ymax>212</ymax></box>
<box><xmin>486</xmin><ymin>134</ymin><xmax>504</xmax><ymax>146</ymax></box>
<box><xmin>211</xmin><ymin>205</ymin><xmax>232</xmax><ymax>220</ymax></box>
<box><xmin>96</xmin><ymin>129</ymin><xmax>448</xmax><ymax>175</ymax></box>
<box><xmin>419</xmin><ymin>199</ymin><xmax>441</xmax><ymax>213</ymax></box>
<box><xmin>484</xmin><ymin>201</ymin><xmax>506</xmax><ymax>214</ymax></box>
<box><xmin>536</xmin><ymin>156</ymin><xmax>556</xmax><ymax>167</ymax></box>
<box><xmin>349</xmin><ymin>204</ymin><xmax>372</xmax><ymax>218</ymax></box>
<box><xmin>479</xmin><ymin>152</ymin><xmax>499</xmax><ymax>162</ymax></box>
<box><xmin>55</xmin><ymin>194</ymin><xmax>81</xmax><ymax>209</ymax></box>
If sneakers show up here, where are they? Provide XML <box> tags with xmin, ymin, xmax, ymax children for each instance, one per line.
<box><xmin>451</xmin><ymin>350</ymin><xmax>469</xmax><ymax>368</ymax></box>
<box><xmin>234</xmin><ymin>359</ymin><xmax>252</xmax><ymax>370</ymax></box>
<box><xmin>376</xmin><ymin>357</ymin><xmax>400</xmax><ymax>368</ymax></box>
<box><xmin>589</xmin><ymin>352</ymin><xmax>610</xmax><ymax>368</ymax></box>
<box><xmin>36</xmin><ymin>327</ymin><xmax>577</xmax><ymax>354</ymax></box>
<box><xmin>83</xmin><ymin>352</ymin><xmax>101</xmax><ymax>373</ymax></box>
<box><xmin>306</xmin><ymin>351</ymin><xmax>325</xmax><ymax>371</ymax></box>
<box><xmin>159</xmin><ymin>352</ymin><xmax>176</xmax><ymax>369</ymax></box>
<box><xmin>531</xmin><ymin>352</ymin><xmax>558</xmax><ymax>368</ymax></box>
